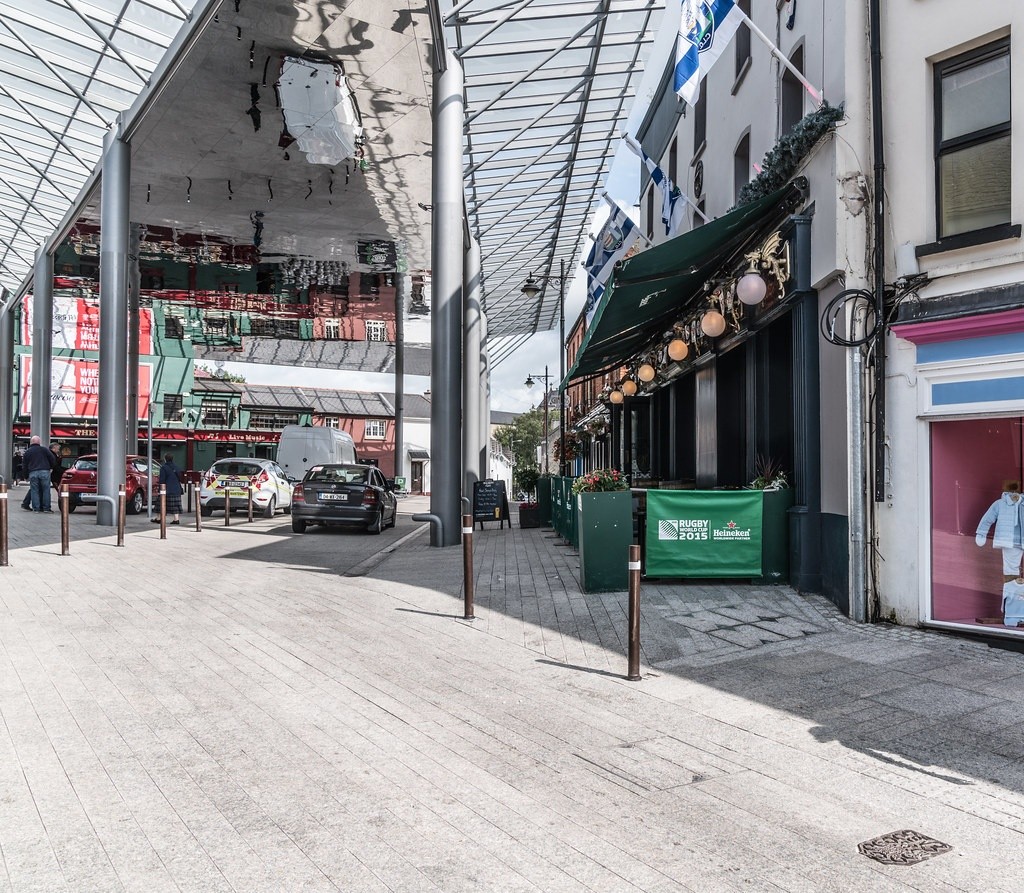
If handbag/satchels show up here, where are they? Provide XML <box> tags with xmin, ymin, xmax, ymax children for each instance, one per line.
<box><xmin>181</xmin><ymin>487</ymin><xmax>184</xmax><ymax>495</ymax></box>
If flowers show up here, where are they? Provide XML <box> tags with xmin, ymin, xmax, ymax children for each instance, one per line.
<box><xmin>519</xmin><ymin>503</ymin><xmax>538</xmax><ymax>509</ymax></box>
<box><xmin>748</xmin><ymin>453</ymin><xmax>791</xmax><ymax>493</ymax></box>
<box><xmin>570</xmin><ymin>467</ymin><xmax>630</xmax><ymax>497</ymax></box>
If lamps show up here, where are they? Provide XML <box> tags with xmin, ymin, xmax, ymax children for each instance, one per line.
<box><xmin>736</xmin><ymin>250</ymin><xmax>767</xmax><ymax>306</ymax></box>
<box><xmin>667</xmin><ymin>326</ymin><xmax>688</xmax><ymax>361</ymax></box>
<box><xmin>702</xmin><ymin>293</ymin><xmax>726</xmax><ymax>338</ymax></box>
<box><xmin>610</xmin><ymin>382</ymin><xmax>623</xmax><ymax>404</ymax></box>
<box><xmin>623</xmin><ymin>372</ymin><xmax>637</xmax><ymax>395</ymax></box>
<box><xmin>638</xmin><ymin>356</ymin><xmax>655</xmax><ymax>382</ymax></box>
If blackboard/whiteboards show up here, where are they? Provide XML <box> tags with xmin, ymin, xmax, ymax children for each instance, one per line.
<box><xmin>473</xmin><ymin>480</ymin><xmax>503</xmax><ymax>521</ymax></box>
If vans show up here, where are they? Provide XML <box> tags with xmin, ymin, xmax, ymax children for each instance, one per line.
<box><xmin>276</xmin><ymin>425</ymin><xmax>359</xmax><ymax>487</ymax></box>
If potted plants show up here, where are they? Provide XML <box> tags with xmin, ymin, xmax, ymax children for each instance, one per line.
<box><xmin>516</xmin><ymin>467</ymin><xmax>541</xmax><ymax>528</ymax></box>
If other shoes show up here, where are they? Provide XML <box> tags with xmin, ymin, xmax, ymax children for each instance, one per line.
<box><xmin>150</xmin><ymin>518</ymin><xmax>160</xmax><ymax>523</ymax></box>
<box><xmin>21</xmin><ymin>504</ymin><xmax>33</xmax><ymax>511</ymax></box>
<box><xmin>170</xmin><ymin>519</ymin><xmax>179</xmax><ymax>525</ymax></box>
<box><xmin>34</xmin><ymin>510</ymin><xmax>39</xmax><ymax>514</ymax></box>
<box><xmin>44</xmin><ymin>509</ymin><xmax>54</xmax><ymax>513</ymax></box>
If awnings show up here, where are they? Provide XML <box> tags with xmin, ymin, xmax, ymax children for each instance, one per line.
<box><xmin>407</xmin><ymin>451</ymin><xmax>430</xmax><ymax>461</ymax></box>
<box><xmin>559</xmin><ymin>176</ymin><xmax>809</xmax><ymax>400</ymax></box>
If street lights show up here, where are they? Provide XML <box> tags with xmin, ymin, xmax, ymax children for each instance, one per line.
<box><xmin>520</xmin><ymin>259</ymin><xmax>576</xmax><ymax>478</ymax></box>
<box><xmin>524</xmin><ymin>366</ymin><xmax>554</xmax><ymax>471</ymax></box>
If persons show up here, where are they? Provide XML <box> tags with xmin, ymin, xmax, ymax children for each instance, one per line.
<box><xmin>22</xmin><ymin>435</ymin><xmax>55</xmax><ymax>513</ymax></box>
<box><xmin>150</xmin><ymin>453</ymin><xmax>184</xmax><ymax>524</ymax></box>
<box><xmin>21</xmin><ymin>442</ymin><xmax>64</xmax><ymax>511</ymax></box>
<box><xmin>13</xmin><ymin>452</ymin><xmax>24</xmax><ymax>481</ymax></box>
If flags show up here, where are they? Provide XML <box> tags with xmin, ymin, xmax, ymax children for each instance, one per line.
<box><xmin>626</xmin><ymin>138</ymin><xmax>688</xmax><ymax>238</ymax></box>
<box><xmin>673</xmin><ymin>0</ymin><xmax>746</xmax><ymax>109</ymax></box>
<box><xmin>586</xmin><ymin>272</ymin><xmax>605</xmax><ymax>333</ymax></box>
<box><xmin>586</xmin><ymin>202</ymin><xmax>641</xmax><ymax>284</ymax></box>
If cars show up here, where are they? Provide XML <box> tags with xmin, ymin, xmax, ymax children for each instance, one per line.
<box><xmin>291</xmin><ymin>464</ymin><xmax>401</xmax><ymax>536</ymax></box>
<box><xmin>57</xmin><ymin>455</ymin><xmax>162</xmax><ymax>515</ymax></box>
<box><xmin>200</xmin><ymin>458</ymin><xmax>296</xmax><ymax>519</ymax></box>
